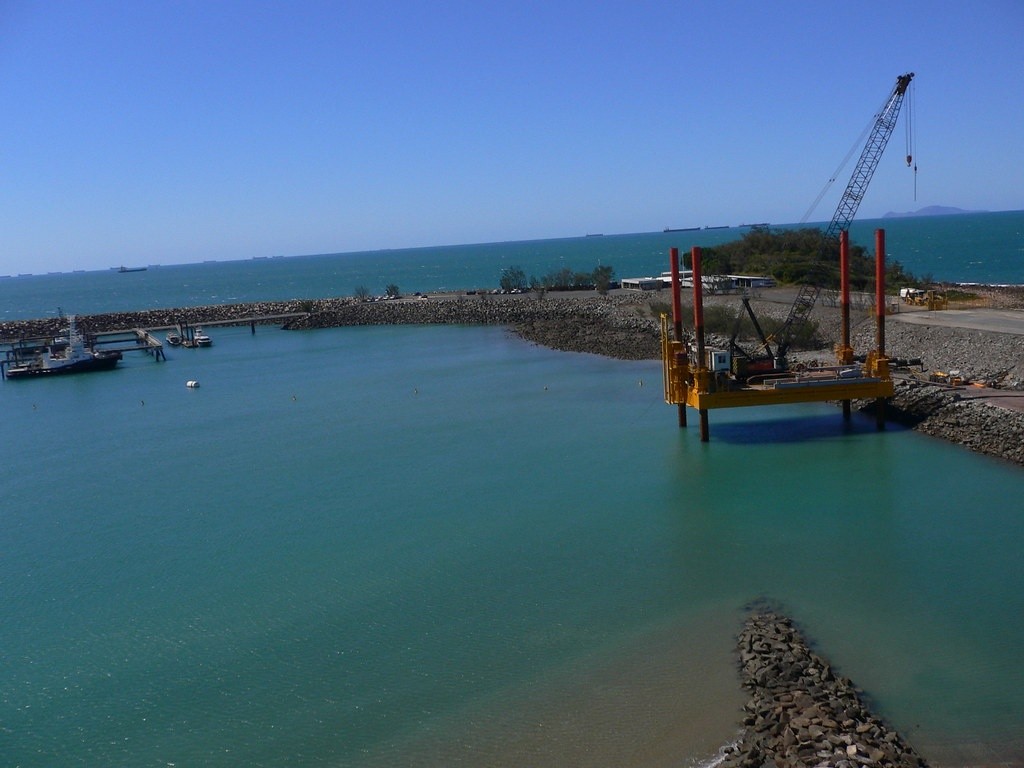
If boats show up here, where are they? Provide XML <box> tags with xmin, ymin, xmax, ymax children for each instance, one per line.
<box><xmin>7</xmin><ymin>320</ymin><xmax>122</xmax><ymax>378</ymax></box>
<box><xmin>166</xmin><ymin>329</ymin><xmax>214</xmax><ymax>349</ymax></box>
<box><xmin>117</xmin><ymin>266</ymin><xmax>147</xmax><ymax>273</ymax></box>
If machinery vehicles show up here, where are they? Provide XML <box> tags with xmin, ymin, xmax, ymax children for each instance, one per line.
<box><xmin>730</xmin><ymin>72</ymin><xmax>916</xmax><ymax>384</ymax></box>
